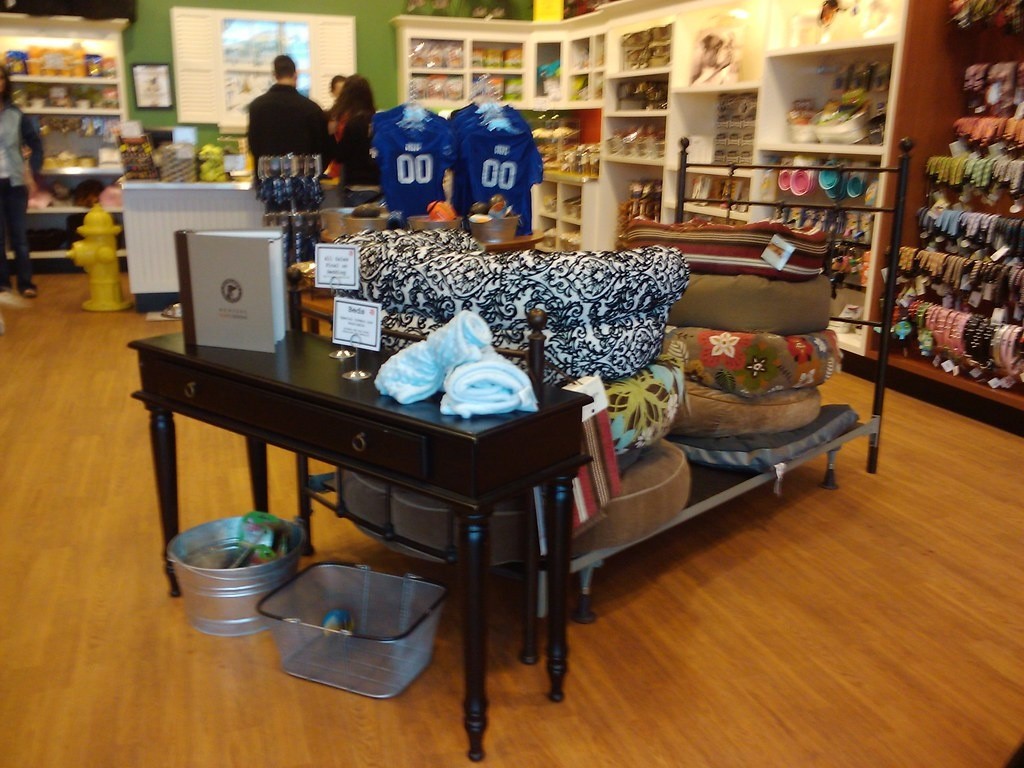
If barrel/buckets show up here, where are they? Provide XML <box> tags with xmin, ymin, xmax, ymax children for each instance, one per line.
<box><xmin>165</xmin><ymin>514</ymin><xmax>305</xmax><ymax>639</ymax></box>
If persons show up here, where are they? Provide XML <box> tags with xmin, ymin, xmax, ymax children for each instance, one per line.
<box><xmin>325</xmin><ymin>75</ymin><xmax>381</xmax><ymax>205</ymax></box>
<box><xmin>247</xmin><ymin>56</ymin><xmax>328</xmax><ymax>265</ymax></box>
<box><xmin>0</xmin><ymin>66</ymin><xmax>44</xmax><ymax>298</ymax></box>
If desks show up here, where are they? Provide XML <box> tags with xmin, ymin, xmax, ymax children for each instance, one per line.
<box><xmin>128</xmin><ymin>328</ymin><xmax>595</xmax><ymax>762</ymax></box>
<box><xmin>481</xmin><ymin>230</ymin><xmax>546</xmax><ymax>253</ymax></box>
<box><xmin>120</xmin><ymin>179</ymin><xmax>342</xmax><ymax>320</ymax></box>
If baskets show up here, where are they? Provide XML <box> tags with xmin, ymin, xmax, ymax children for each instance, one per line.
<box><xmin>257</xmin><ymin>561</ymin><xmax>447</xmax><ymax>698</ymax></box>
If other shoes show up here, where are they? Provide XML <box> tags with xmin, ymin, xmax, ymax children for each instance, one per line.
<box><xmin>16</xmin><ymin>282</ymin><xmax>37</xmax><ymax>299</ymax></box>
<box><xmin>0</xmin><ymin>282</ymin><xmax>12</xmax><ymax>292</ymax></box>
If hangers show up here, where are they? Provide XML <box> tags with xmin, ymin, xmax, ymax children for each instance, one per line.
<box><xmin>473</xmin><ymin>75</ymin><xmax>517</xmax><ymax>134</ymax></box>
<box><xmin>397</xmin><ymin>81</ymin><xmax>432</xmax><ymax>130</ymax></box>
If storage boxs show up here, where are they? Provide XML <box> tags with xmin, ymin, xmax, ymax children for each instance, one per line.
<box><xmin>256</xmin><ymin>561</ymin><xmax>447</xmax><ymax>698</ymax></box>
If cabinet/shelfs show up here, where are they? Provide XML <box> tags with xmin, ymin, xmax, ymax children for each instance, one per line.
<box><xmin>389</xmin><ymin>0</ymin><xmax>1023</xmax><ymax>434</ymax></box>
<box><xmin>0</xmin><ymin>11</ymin><xmax>132</xmax><ymax>265</ymax></box>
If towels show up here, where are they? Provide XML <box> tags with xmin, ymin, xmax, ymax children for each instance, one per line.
<box><xmin>373</xmin><ymin>311</ymin><xmax>492</xmax><ymax>405</ymax></box>
<box><xmin>440</xmin><ymin>345</ymin><xmax>540</xmax><ymax>419</ymax></box>
